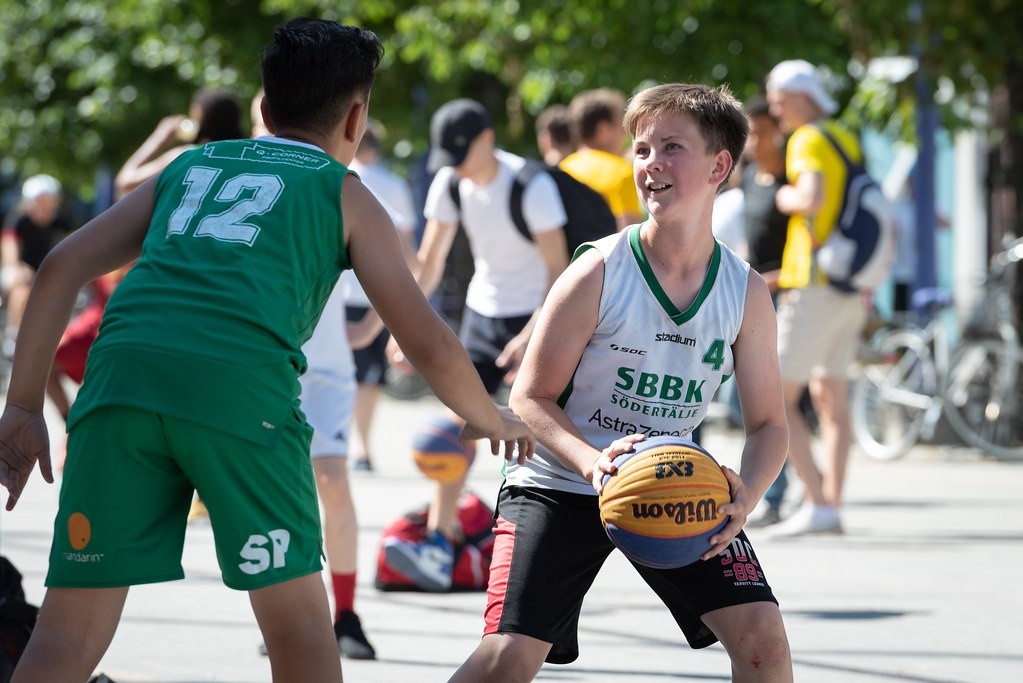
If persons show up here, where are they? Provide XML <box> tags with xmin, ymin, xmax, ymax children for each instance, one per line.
<box><xmin>0</xmin><ymin>55</ymin><xmax>869</xmax><ymax>664</ymax></box>
<box><xmin>448</xmin><ymin>84</ymin><xmax>796</xmax><ymax>683</ymax></box>
<box><xmin>0</xmin><ymin>17</ymin><xmax>535</xmax><ymax>683</ymax></box>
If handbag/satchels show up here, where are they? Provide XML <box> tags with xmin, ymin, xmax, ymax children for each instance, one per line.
<box><xmin>373</xmin><ymin>492</ymin><xmax>496</xmax><ymax>592</ymax></box>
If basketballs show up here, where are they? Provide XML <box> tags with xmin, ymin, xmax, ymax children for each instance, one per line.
<box><xmin>597</xmin><ymin>436</ymin><xmax>731</xmax><ymax>568</ymax></box>
<box><xmin>411</xmin><ymin>414</ymin><xmax>476</xmax><ymax>481</ymax></box>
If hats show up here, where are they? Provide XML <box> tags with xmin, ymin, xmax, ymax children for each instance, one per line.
<box><xmin>768</xmin><ymin>59</ymin><xmax>840</xmax><ymax>116</ymax></box>
<box><xmin>425</xmin><ymin>98</ymin><xmax>491</xmax><ymax>172</ymax></box>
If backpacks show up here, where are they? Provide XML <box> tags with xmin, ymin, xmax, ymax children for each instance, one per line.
<box><xmin>810</xmin><ymin>118</ymin><xmax>895</xmax><ymax>294</ymax></box>
<box><xmin>447</xmin><ymin>158</ymin><xmax>618</xmax><ymax>259</ymax></box>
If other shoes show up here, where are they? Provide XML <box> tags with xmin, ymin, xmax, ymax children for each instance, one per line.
<box><xmin>766</xmin><ymin>503</ymin><xmax>839</xmax><ymax>536</ymax></box>
<box><xmin>385</xmin><ymin>528</ymin><xmax>454</xmax><ymax>591</ymax></box>
<box><xmin>354</xmin><ymin>457</ymin><xmax>371</xmax><ymax>471</ymax></box>
<box><xmin>746</xmin><ymin>509</ymin><xmax>780</xmax><ymax>529</ymax></box>
<box><xmin>333</xmin><ymin>608</ymin><xmax>377</xmax><ymax>659</ymax></box>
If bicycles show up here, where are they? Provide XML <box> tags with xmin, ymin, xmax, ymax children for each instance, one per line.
<box><xmin>849</xmin><ymin>234</ymin><xmax>1022</xmax><ymax>463</ymax></box>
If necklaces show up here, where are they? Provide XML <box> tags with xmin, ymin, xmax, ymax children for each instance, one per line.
<box><xmin>278</xmin><ymin>133</ymin><xmax>315</xmax><ymax>143</ymax></box>
